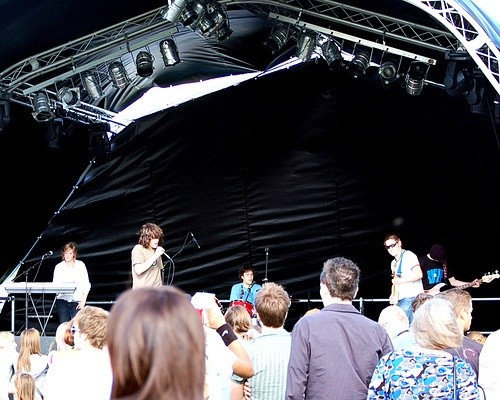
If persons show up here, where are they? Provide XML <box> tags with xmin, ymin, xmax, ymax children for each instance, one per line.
<box><xmin>384</xmin><ymin>234</ymin><xmax>424</xmax><ymax>325</ymax></box>
<box><xmin>0</xmin><ymin>282</ymin><xmax>500</xmax><ymax>400</ymax></box>
<box><xmin>285</xmin><ymin>257</ymin><xmax>394</xmax><ymax>400</ymax></box>
<box><xmin>418</xmin><ymin>244</ymin><xmax>453</xmax><ymax>291</ymax></box>
<box><xmin>229</xmin><ymin>266</ymin><xmax>261</xmax><ymax>318</ymax></box>
<box><xmin>131</xmin><ymin>223</ymin><xmax>165</xmax><ymax>288</ymax></box>
<box><xmin>53</xmin><ymin>242</ymin><xmax>91</xmax><ymax>324</ymax></box>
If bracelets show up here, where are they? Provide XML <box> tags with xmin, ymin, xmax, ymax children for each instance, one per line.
<box><xmin>216</xmin><ymin>323</ymin><xmax>238</xmax><ymax>346</ymax></box>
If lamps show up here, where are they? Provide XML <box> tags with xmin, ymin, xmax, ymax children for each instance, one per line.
<box><xmin>104</xmin><ymin>57</ymin><xmax>129</xmax><ymax>89</ymax></box>
<box><xmin>267</xmin><ymin>18</ymin><xmax>291</xmax><ymax>52</ymax></box>
<box><xmin>161</xmin><ymin>0</ymin><xmax>233</xmax><ymax>41</ymax></box>
<box><xmin>159</xmin><ymin>35</ymin><xmax>181</xmax><ymax>66</ymax></box>
<box><xmin>55</xmin><ymin>77</ymin><xmax>82</xmax><ymax>109</ymax></box>
<box><xmin>0</xmin><ymin>100</ymin><xmax>11</xmax><ymax>131</ymax></box>
<box><xmin>30</xmin><ymin>87</ymin><xmax>55</xmax><ymax>123</ymax></box>
<box><xmin>80</xmin><ymin>66</ymin><xmax>102</xmax><ymax>99</ymax></box>
<box><xmin>349</xmin><ymin>41</ymin><xmax>373</xmax><ymax>73</ymax></box>
<box><xmin>88</xmin><ymin>132</ymin><xmax>111</xmax><ymax>159</ymax></box>
<box><xmin>130</xmin><ymin>45</ymin><xmax>155</xmax><ymax>77</ymax></box>
<box><xmin>442</xmin><ymin>58</ymin><xmax>500</xmax><ymax>123</ymax></box>
<box><xmin>404</xmin><ymin>57</ymin><xmax>430</xmax><ymax>96</ymax></box>
<box><xmin>378</xmin><ymin>50</ymin><xmax>403</xmax><ymax>82</ymax></box>
<box><xmin>321</xmin><ymin>34</ymin><xmax>343</xmax><ymax>66</ymax></box>
<box><xmin>295</xmin><ymin>27</ymin><xmax>316</xmax><ymax>61</ymax></box>
<box><xmin>48</xmin><ymin>118</ymin><xmax>64</xmax><ymax>149</ymax></box>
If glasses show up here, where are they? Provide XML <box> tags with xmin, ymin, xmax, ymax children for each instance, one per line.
<box><xmin>70</xmin><ymin>326</ymin><xmax>81</xmax><ymax>335</ymax></box>
<box><xmin>385</xmin><ymin>242</ymin><xmax>398</xmax><ymax>249</ymax></box>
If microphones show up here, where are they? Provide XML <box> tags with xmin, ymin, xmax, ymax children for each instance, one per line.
<box><xmin>248</xmin><ymin>281</ymin><xmax>256</xmax><ymax>289</ymax></box>
<box><xmin>44</xmin><ymin>251</ymin><xmax>53</xmax><ymax>256</ymax></box>
<box><xmin>190</xmin><ymin>232</ymin><xmax>200</xmax><ymax>248</ymax></box>
<box><xmin>163</xmin><ymin>253</ymin><xmax>172</xmax><ymax>260</ymax></box>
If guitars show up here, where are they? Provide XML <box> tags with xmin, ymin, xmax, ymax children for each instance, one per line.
<box><xmin>426</xmin><ymin>270</ymin><xmax>500</xmax><ymax>297</ymax></box>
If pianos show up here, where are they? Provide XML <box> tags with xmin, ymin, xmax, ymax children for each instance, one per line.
<box><xmin>4</xmin><ymin>282</ymin><xmax>76</xmax><ymax>336</ymax></box>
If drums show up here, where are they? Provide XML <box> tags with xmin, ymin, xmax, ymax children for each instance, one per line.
<box><xmin>229</xmin><ymin>300</ymin><xmax>253</xmax><ymax>318</ymax></box>
